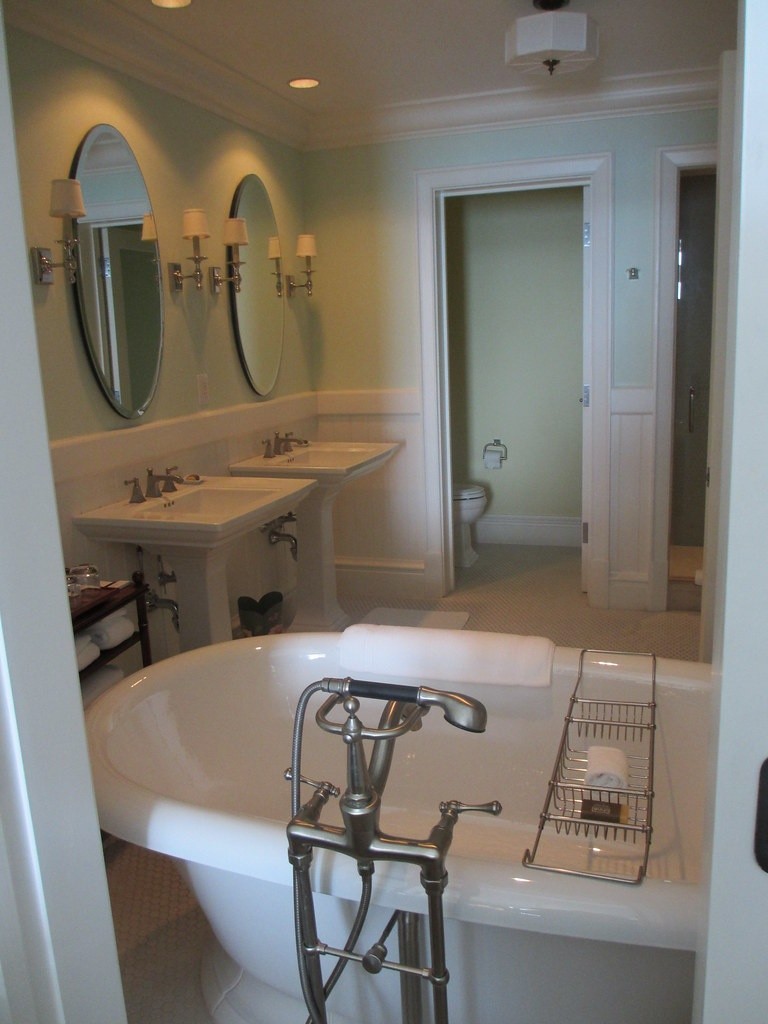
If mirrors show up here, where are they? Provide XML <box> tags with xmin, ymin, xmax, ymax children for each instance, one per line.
<box><xmin>230</xmin><ymin>174</ymin><xmax>285</xmax><ymax>396</ymax></box>
<box><xmin>73</xmin><ymin>124</ymin><xmax>163</xmax><ymax>419</ymax></box>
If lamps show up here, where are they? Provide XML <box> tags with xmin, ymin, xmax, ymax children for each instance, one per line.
<box><xmin>286</xmin><ymin>234</ymin><xmax>317</xmax><ymax>296</ymax></box>
<box><xmin>140</xmin><ymin>213</ymin><xmax>157</xmax><ymax>241</ymax></box>
<box><xmin>210</xmin><ymin>218</ymin><xmax>251</xmax><ymax>294</ymax></box>
<box><xmin>505</xmin><ymin>0</ymin><xmax>599</xmax><ymax>75</ymax></box>
<box><xmin>268</xmin><ymin>237</ymin><xmax>282</xmax><ymax>298</ymax></box>
<box><xmin>167</xmin><ymin>208</ymin><xmax>210</xmax><ymax>293</ymax></box>
<box><xmin>30</xmin><ymin>179</ymin><xmax>85</xmax><ymax>283</ymax></box>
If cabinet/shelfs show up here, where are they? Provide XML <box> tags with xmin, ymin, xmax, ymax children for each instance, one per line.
<box><xmin>67</xmin><ymin>571</ymin><xmax>153</xmax><ymax>709</ymax></box>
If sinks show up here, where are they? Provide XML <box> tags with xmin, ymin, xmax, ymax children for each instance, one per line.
<box><xmin>71</xmin><ymin>474</ymin><xmax>320</xmax><ymax>552</ymax></box>
<box><xmin>228</xmin><ymin>440</ymin><xmax>400</xmax><ymax>487</ymax></box>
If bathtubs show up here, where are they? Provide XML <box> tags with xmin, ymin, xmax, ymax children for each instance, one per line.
<box><xmin>84</xmin><ymin>632</ymin><xmax>713</xmax><ymax>1024</ymax></box>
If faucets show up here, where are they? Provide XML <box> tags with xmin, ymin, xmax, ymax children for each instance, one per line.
<box><xmin>268</xmin><ymin>530</ymin><xmax>298</xmax><ymax>563</ymax></box>
<box><xmin>144</xmin><ymin>466</ymin><xmax>185</xmax><ymax>499</ymax></box>
<box><xmin>152</xmin><ymin>598</ymin><xmax>181</xmax><ymax>633</ymax></box>
<box><xmin>274</xmin><ymin>431</ymin><xmax>305</xmax><ymax>455</ymax></box>
<box><xmin>367</xmin><ymin>695</ymin><xmax>433</xmax><ymax>798</ymax></box>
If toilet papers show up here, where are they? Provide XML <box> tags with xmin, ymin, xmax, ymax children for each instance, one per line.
<box><xmin>484</xmin><ymin>449</ymin><xmax>503</xmax><ymax>469</ymax></box>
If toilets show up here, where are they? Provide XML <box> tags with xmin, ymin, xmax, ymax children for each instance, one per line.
<box><xmin>452</xmin><ymin>482</ymin><xmax>489</xmax><ymax>568</ymax></box>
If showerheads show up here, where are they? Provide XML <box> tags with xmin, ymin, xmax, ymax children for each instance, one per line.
<box><xmin>320</xmin><ymin>676</ymin><xmax>489</xmax><ymax>733</ymax></box>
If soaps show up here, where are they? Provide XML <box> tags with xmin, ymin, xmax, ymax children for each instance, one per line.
<box><xmin>579</xmin><ymin>798</ymin><xmax>631</xmax><ymax>824</ymax></box>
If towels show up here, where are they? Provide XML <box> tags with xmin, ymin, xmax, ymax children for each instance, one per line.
<box><xmin>583</xmin><ymin>745</ymin><xmax>629</xmax><ymax>792</ymax></box>
<box><xmin>315</xmin><ymin>623</ymin><xmax>556</xmax><ymax>687</ymax></box>
<box><xmin>74</xmin><ymin>607</ymin><xmax>135</xmax><ymax>711</ymax></box>
